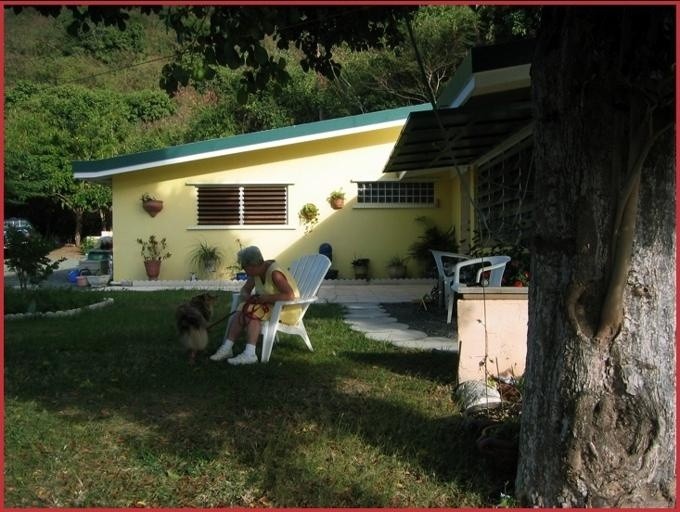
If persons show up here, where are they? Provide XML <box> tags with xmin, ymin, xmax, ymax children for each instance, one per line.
<box><xmin>209</xmin><ymin>245</ymin><xmax>302</xmax><ymax>366</ymax></box>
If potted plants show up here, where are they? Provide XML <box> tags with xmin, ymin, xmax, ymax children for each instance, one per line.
<box><xmin>136</xmin><ymin>235</ymin><xmax>173</xmax><ymax>281</ymax></box>
<box><xmin>297</xmin><ymin>202</ymin><xmax>320</xmax><ymax>240</ymax></box>
<box><xmin>225</xmin><ymin>237</ymin><xmax>249</xmax><ymax>280</ymax></box>
<box><xmin>183</xmin><ymin>239</ymin><xmax>225</xmax><ymax>280</ymax></box>
<box><xmin>326</xmin><ymin>191</ymin><xmax>346</xmax><ymax>210</ymax></box>
<box><xmin>383</xmin><ymin>254</ymin><xmax>412</xmax><ymax>280</ymax></box>
<box><xmin>138</xmin><ymin>190</ymin><xmax>163</xmax><ymax>218</ymax></box>
<box><xmin>350</xmin><ymin>255</ymin><xmax>370</xmax><ymax>276</ymax></box>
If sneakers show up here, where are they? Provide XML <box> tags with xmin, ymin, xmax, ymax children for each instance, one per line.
<box><xmin>210</xmin><ymin>347</ymin><xmax>237</xmax><ymax>362</ymax></box>
<box><xmin>229</xmin><ymin>353</ymin><xmax>258</xmax><ymax>365</ymax></box>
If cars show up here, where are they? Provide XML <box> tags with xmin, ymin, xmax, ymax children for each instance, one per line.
<box><xmin>3</xmin><ymin>216</ymin><xmax>36</xmax><ymax>255</ymax></box>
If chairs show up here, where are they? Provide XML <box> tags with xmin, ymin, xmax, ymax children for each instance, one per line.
<box><xmin>431</xmin><ymin>250</ymin><xmax>473</xmax><ymax>311</ymax></box>
<box><xmin>446</xmin><ymin>255</ymin><xmax>511</xmax><ymax>324</ymax></box>
<box><xmin>223</xmin><ymin>253</ymin><xmax>332</xmax><ymax>363</ymax></box>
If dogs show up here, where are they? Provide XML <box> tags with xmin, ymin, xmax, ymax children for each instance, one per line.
<box><xmin>175</xmin><ymin>292</ymin><xmax>221</xmax><ymax>363</ymax></box>
